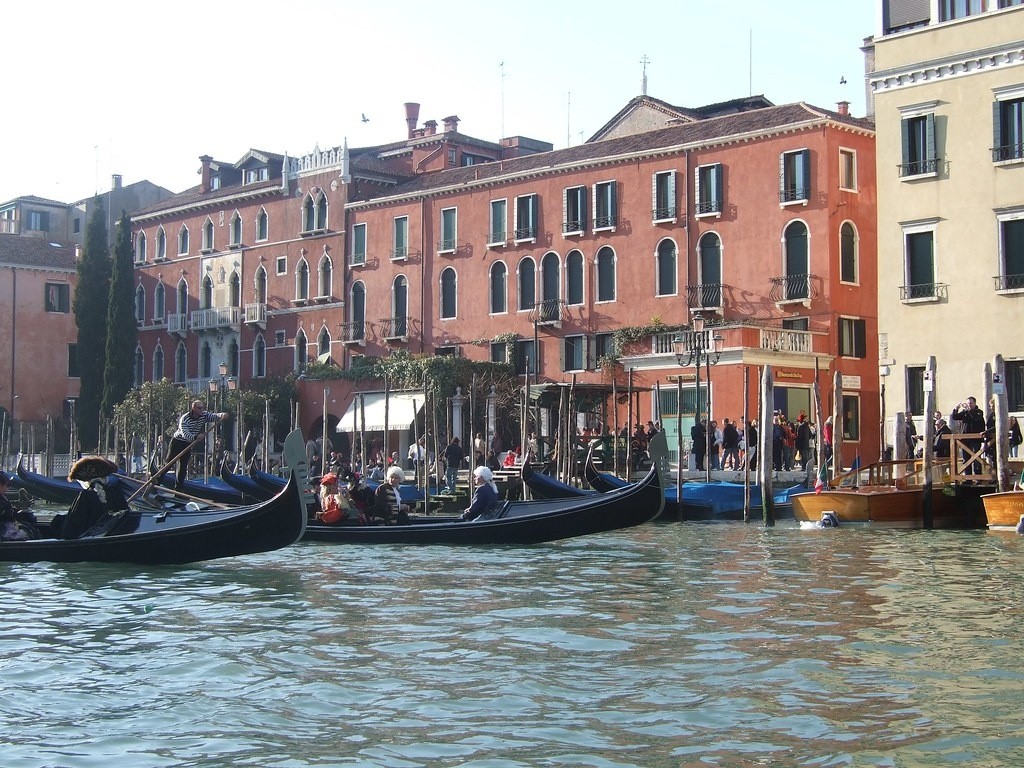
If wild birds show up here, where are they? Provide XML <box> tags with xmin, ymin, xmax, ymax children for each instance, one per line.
<box><xmin>839</xmin><ymin>76</ymin><xmax>847</xmax><ymax>85</ymax></box>
<box><xmin>361</xmin><ymin>112</ymin><xmax>370</xmax><ymax>124</ymax></box>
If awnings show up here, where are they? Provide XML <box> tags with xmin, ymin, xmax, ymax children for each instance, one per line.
<box><xmin>336</xmin><ymin>391</ymin><xmax>425</xmax><ymax>433</ymax></box>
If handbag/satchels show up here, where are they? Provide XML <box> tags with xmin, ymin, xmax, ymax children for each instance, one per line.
<box><xmin>0</xmin><ymin>521</ymin><xmax>29</xmax><ymax>541</ymax></box>
<box><xmin>322</xmin><ymin>507</ymin><xmax>344</xmax><ymax>523</ymax></box>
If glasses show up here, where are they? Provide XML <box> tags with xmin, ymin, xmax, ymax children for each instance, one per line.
<box><xmin>196</xmin><ymin>407</ymin><xmax>203</xmax><ymax>409</ymax></box>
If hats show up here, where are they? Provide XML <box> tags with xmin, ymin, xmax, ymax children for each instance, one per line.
<box><xmin>798</xmin><ymin>414</ymin><xmax>807</xmax><ymax>422</ymax></box>
<box><xmin>646</xmin><ymin>421</ymin><xmax>652</xmax><ymax>424</ymax></box>
<box><xmin>322</xmin><ymin>473</ymin><xmax>337</xmax><ymax>485</ymax></box>
<box><xmin>451</xmin><ymin>436</ymin><xmax>461</xmax><ymax>443</ymax></box>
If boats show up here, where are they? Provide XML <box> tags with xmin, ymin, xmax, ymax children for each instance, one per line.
<box><xmin>0</xmin><ymin>426</ymin><xmax>444</xmax><ymax>562</ymax></box>
<box><xmin>248</xmin><ymin>428</ymin><xmax>672</xmax><ymax>539</ymax></box>
<box><xmin>521</xmin><ymin>442</ymin><xmax>862</xmax><ymax>521</ymax></box>
<box><xmin>789</xmin><ymin>457</ymin><xmax>993</xmax><ymax>532</ymax></box>
<box><xmin>979</xmin><ymin>491</ymin><xmax>1024</xmax><ymax>531</ymax></box>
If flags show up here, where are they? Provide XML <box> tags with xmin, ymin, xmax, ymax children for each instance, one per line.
<box><xmin>849</xmin><ymin>457</ymin><xmax>861</xmax><ymax>473</ymax></box>
<box><xmin>815</xmin><ymin>463</ymin><xmax>826</xmax><ymax>496</ymax></box>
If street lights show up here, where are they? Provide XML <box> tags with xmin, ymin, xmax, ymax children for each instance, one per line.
<box><xmin>209</xmin><ymin>362</ymin><xmax>238</xmax><ymax>461</ymax></box>
<box><xmin>670</xmin><ymin>312</ymin><xmax>726</xmax><ymax>470</ymax></box>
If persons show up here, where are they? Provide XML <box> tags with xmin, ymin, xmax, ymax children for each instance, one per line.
<box><xmin>691</xmin><ymin>414</ymin><xmax>833</xmax><ymax>472</ymax></box>
<box><xmin>374</xmin><ymin>466</ymin><xmax>412</xmax><ymax>526</ymax></box>
<box><xmin>305</xmin><ymin>434</ymin><xmax>400</xmax><ymax>480</ymax></box>
<box><xmin>311</xmin><ymin>472</ymin><xmax>375</xmax><ymax>525</ymax></box>
<box><xmin>131</xmin><ymin>431</ymin><xmax>144</xmax><ymax>473</ymax></box>
<box><xmin>406</xmin><ymin>429</ymin><xmax>464</xmax><ymax>494</ymax></box>
<box><xmin>157</xmin><ymin>436</ymin><xmax>168</xmax><ymax>465</ymax></box>
<box><xmin>0</xmin><ymin>470</ymin><xmax>40</xmax><ymax>541</ymax></box>
<box><xmin>575</xmin><ymin>421</ymin><xmax>659</xmax><ymax>471</ymax></box>
<box><xmin>474</xmin><ymin>431</ymin><xmax>557</xmax><ymax>472</ymax></box>
<box><xmin>152</xmin><ymin>399</ymin><xmax>229</xmax><ymax>491</ymax></box>
<box><xmin>51</xmin><ymin>456</ymin><xmax>128</xmax><ymax>539</ymax></box>
<box><xmin>878</xmin><ymin>397</ymin><xmax>1022</xmax><ymax>485</ymax></box>
<box><xmin>458</xmin><ymin>466</ymin><xmax>499</xmax><ymax>521</ymax></box>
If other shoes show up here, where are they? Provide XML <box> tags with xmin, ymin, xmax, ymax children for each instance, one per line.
<box><xmin>447</xmin><ymin>490</ymin><xmax>456</xmax><ymax>495</ymax></box>
<box><xmin>175</xmin><ymin>486</ymin><xmax>192</xmax><ymax>494</ymax></box>
<box><xmin>698</xmin><ymin>466</ymin><xmax>806</xmax><ymax>472</ymax></box>
